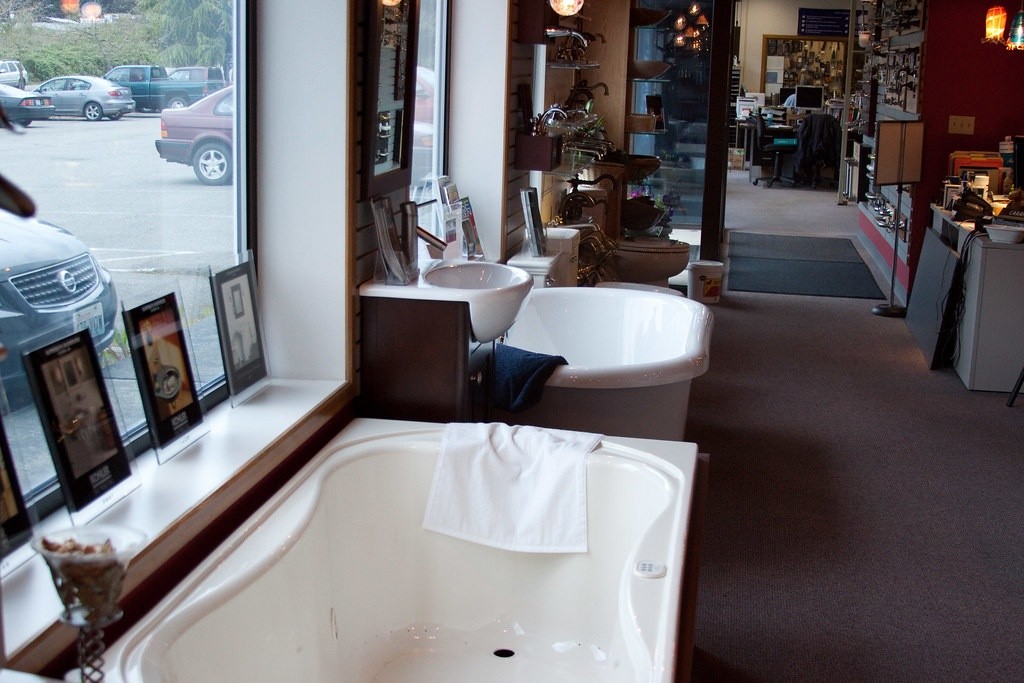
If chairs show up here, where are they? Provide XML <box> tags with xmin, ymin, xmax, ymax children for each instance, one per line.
<box><xmin>796</xmin><ymin>113</ymin><xmax>843</xmax><ymax>189</ymax></box>
<box><xmin>752</xmin><ymin>107</ymin><xmax>798</xmax><ymax>189</ymax></box>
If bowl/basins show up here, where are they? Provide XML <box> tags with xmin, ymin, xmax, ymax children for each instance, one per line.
<box><xmin>983</xmin><ymin>224</ymin><xmax>1024</xmax><ymax>244</ymax></box>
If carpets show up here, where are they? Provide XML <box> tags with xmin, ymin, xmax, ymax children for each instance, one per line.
<box><xmin>727</xmin><ymin>256</ymin><xmax>888</xmax><ymax>300</ymax></box>
<box><xmin>727</xmin><ymin>231</ymin><xmax>865</xmax><ymax>262</ymax></box>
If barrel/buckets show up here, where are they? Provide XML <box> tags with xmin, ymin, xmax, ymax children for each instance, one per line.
<box><xmin>686</xmin><ymin>260</ymin><xmax>724</xmax><ymax>304</ymax></box>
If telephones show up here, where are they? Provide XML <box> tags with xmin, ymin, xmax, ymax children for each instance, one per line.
<box><xmin>953</xmin><ymin>192</ymin><xmax>993</xmax><ymax>221</ymax></box>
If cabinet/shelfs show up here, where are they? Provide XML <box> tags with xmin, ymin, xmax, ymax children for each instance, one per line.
<box><xmin>926</xmin><ymin>201</ymin><xmax>1024</xmax><ymax>394</ymax></box>
<box><xmin>624</xmin><ymin>24</ymin><xmax>672</xmax><ymax>135</ymax></box>
<box><xmin>539</xmin><ymin>60</ymin><xmax>616</xmax><ymax>182</ymax></box>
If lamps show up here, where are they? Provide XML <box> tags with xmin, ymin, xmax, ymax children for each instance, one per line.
<box><xmin>979</xmin><ymin>0</ymin><xmax>1006</xmax><ymax>46</ymax></box>
<box><xmin>1006</xmin><ymin>0</ymin><xmax>1024</xmax><ymax>51</ymax></box>
<box><xmin>660</xmin><ymin>0</ymin><xmax>711</xmax><ymax>59</ymax></box>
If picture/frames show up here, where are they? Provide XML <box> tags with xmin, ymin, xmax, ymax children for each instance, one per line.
<box><xmin>116</xmin><ymin>293</ymin><xmax>208</xmax><ymax>446</ymax></box>
<box><xmin>0</xmin><ymin>421</ymin><xmax>30</xmax><ymax>555</ymax></box>
<box><xmin>18</xmin><ymin>330</ymin><xmax>136</xmax><ymax>513</ymax></box>
<box><xmin>211</xmin><ymin>263</ymin><xmax>271</xmax><ymax>396</ymax></box>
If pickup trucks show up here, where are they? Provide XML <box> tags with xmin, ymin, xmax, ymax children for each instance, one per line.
<box><xmin>101</xmin><ymin>65</ymin><xmax>223</xmax><ymax>112</ymax></box>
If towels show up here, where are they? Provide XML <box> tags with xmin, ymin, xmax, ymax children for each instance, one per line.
<box><xmin>422</xmin><ymin>422</ymin><xmax>606</xmax><ymax>555</ymax></box>
<box><xmin>476</xmin><ymin>343</ymin><xmax>569</xmax><ymax>429</ymax></box>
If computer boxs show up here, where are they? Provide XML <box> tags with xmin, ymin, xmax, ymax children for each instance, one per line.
<box><xmin>796</xmin><ymin>119</ymin><xmax>804</xmax><ymax>125</ymax></box>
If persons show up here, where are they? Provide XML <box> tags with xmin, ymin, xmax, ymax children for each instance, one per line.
<box><xmin>783</xmin><ymin>91</ymin><xmax>797</xmax><ymax>108</ymax></box>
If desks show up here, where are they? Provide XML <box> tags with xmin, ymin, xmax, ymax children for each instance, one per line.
<box><xmin>740</xmin><ymin>123</ymin><xmax>785</xmax><ymax>170</ymax></box>
<box><xmin>749</xmin><ymin>124</ymin><xmax>798</xmax><ymax>180</ymax></box>
<box><xmin>735</xmin><ymin>118</ymin><xmax>786</xmax><ymax>122</ymax></box>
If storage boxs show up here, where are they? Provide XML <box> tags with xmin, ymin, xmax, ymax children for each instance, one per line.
<box><xmin>728</xmin><ymin>147</ymin><xmax>744</xmax><ymax>170</ymax></box>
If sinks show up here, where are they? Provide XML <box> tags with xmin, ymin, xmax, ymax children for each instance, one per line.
<box><xmin>358</xmin><ymin>257</ymin><xmax>535</xmax><ymax>343</ymax></box>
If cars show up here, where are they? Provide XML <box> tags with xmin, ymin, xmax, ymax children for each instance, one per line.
<box><xmin>168</xmin><ymin>67</ymin><xmax>224</xmax><ymax>81</ymax></box>
<box><xmin>155</xmin><ymin>85</ymin><xmax>434</xmax><ymax>185</ymax></box>
<box><xmin>0</xmin><ymin>84</ymin><xmax>55</xmax><ymax>126</ymax></box>
<box><xmin>0</xmin><ymin>209</ymin><xmax>118</xmax><ymax>417</ymax></box>
<box><xmin>0</xmin><ymin>61</ymin><xmax>28</xmax><ymax>88</ymax></box>
<box><xmin>29</xmin><ymin>76</ymin><xmax>136</xmax><ymax>121</ymax></box>
<box><xmin>413</xmin><ymin>65</ymin><xmax>435</xmax><ymax>124</ymax></box>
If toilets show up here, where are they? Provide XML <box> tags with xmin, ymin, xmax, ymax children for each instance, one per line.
<box><xmin>568</xmin><ymin>184</ymin><xmax>690</xmax><ymax>287</ymax></box>
<box><xmin>506</xmin><ymin>247</ymin><xmax>565</xmax><ymax>290</ymax></box>
<box><xmin>528</xmin><ymin>226</ymin><xmax>683</xmax><ymax>296</ymax></box>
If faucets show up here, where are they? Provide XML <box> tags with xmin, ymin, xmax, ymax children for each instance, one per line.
<box><xmin>399</xmin><ymin>202</ymin><xmax>448</xmax><ymax>280</ymax></box>
<box><xmin>555</xmin><ymin>31</ymin><xmax>620</xmax><ymax>287</ymax></box>
<box><xmin>529</xmin><ymin>106</ymin><xmax>568</xmax><ymax>136</ymax></box>
<box><xmin>545</xmin><ymin>25</ymin><xmax>588</xmax><ymax>49</ymax></box>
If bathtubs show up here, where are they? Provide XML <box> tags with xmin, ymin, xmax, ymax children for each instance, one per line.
<box><xmin>64</xmin><ymin>417</ymin><xmax>698</xmax><ymax>683</ymax></box>
<box><xmin>492</xmin><ymin>286</ymin><xmax>714</xmax><ymax>442</ymax></box>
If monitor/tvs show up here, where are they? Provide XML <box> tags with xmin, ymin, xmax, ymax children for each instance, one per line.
<box><xmin>780</xmin><ymin>85</ymin><xmax>824</xmax><ymax>115</ymax></box>
